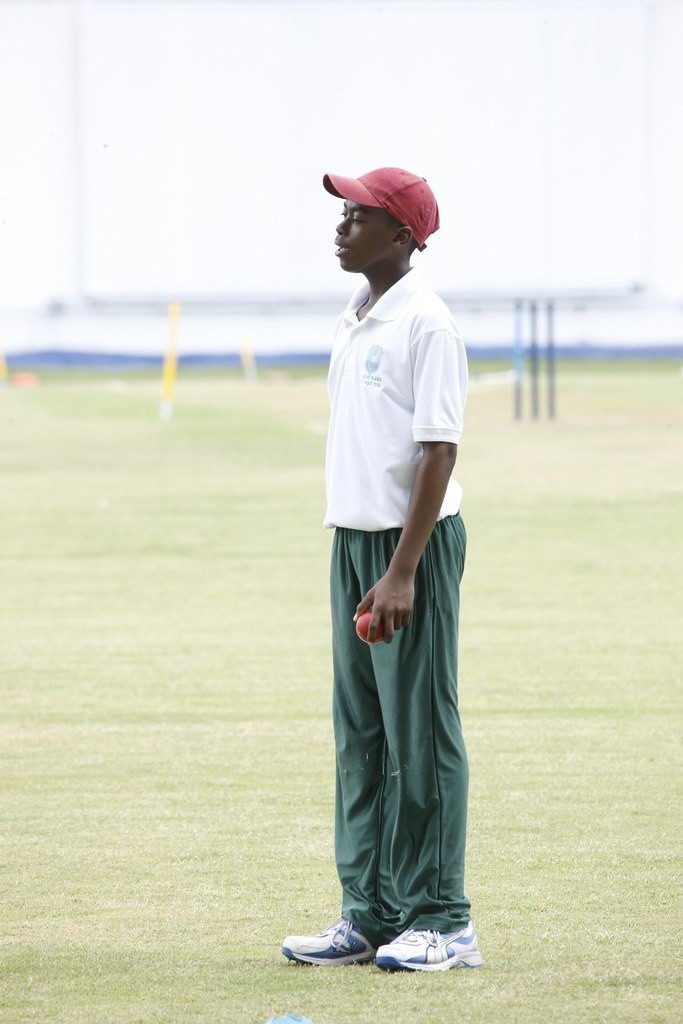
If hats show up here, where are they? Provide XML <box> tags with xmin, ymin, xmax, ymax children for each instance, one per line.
<box><xmin>324</xmin><ymin>166</ymin><xmax>440</xmax><ymax>252</ymax></box>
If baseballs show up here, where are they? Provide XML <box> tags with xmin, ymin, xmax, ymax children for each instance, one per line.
<box><xmin>355</xmin><ymin>610</ymin><xmax>386</xmax><ymax>644</ymax></box>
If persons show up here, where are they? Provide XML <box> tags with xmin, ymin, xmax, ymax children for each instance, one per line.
<box><xmin>283</xmin><ymin>166</ymin><xmax>486</xmax><ymax>972</ymax></box>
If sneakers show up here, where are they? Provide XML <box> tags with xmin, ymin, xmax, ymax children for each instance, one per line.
<box><xmin>376</xmin><ymin>917</ymin><xmax>480</xmax><ymax>973</ymax></box>
<box><xmin>283</xmin><ymin>910</ymin><xmax>376</xmax><ymax>966</ymax></box>
